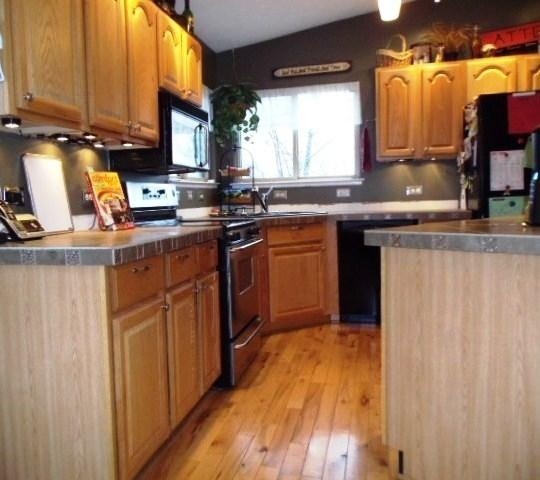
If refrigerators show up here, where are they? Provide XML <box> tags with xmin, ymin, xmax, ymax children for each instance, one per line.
<box><xmin>462</xmin><ymin>88</ymin><xmax>539</xmax><ymax>221</ymax></box>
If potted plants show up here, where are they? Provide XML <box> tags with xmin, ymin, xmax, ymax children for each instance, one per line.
<box><xmin>209</xmin><ymin>75</ymin><xmax>262</xmax><ymax>151</ymax></box>
<box><xmin>419</xmin><ymin>22</ymin><xmax>477</xmax><ymax>62</ymax></box>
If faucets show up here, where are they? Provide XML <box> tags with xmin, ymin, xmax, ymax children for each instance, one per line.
<box><xmin>261</xmin><ymin>187</ymin><xmax>274</xmax><ymax>213</ymax></box>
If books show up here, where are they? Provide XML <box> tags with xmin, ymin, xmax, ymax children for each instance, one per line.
<box><xmin>81</xmin><ymin>171</ymin><xmax>135</xmax><ymax>230</ymax></box>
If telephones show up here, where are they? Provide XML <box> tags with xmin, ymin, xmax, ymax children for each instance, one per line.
<box><xmin>0</xmin><ymin>184</ymin><xmax>47</xmax><ymax>242</ymax></box>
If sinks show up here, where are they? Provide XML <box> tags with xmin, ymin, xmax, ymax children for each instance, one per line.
<box><xmin>249</xmin><ymin>211</ymin><xmax>327</xmax><ymax>217</ymax></box>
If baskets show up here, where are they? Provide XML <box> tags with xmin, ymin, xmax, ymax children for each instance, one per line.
<box><xmin>375</xmin><ymin>33</ymin><xmax>415</xmax><ymax>68</ymax></box>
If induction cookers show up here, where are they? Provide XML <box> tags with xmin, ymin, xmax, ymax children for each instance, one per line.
<box><xmin>119</xmin><ymin>174</ymin><xmax>258</xmax><ymax>244</ymax></box>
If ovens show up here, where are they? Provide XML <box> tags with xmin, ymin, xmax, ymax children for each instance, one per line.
<box><xmin>111</xmin><ymin>104</ymin><xmax>211</xmax><ymax>172</ymax></box>
<box><xmin>220</xmin><ymin>237</ymin><xmax>267</xmax><ymax>388</ymax></box>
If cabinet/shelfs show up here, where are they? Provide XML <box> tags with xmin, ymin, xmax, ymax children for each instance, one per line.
<box><xmin>264</xmin><ymin>219</ymin><xmax>331</xmax><ymax>334</ymax></box>
<box><xmin>164</xmin><ymin>228</ymin><xmax>227</xmax><ymax>435</ymax></box>
<box><xmin>465</xmin><ymin>54</ymin><xmax>539</xmax><ymax>105</ymax></box>
<box><xmin>160</xmin><ymin>9</ymin><xmax>203</xmax><ymax>107</ymax></box>
<box><xmin>376</xmin><ymin>60</ymin><xmax>465</xmax><ymax>163</ymax></box>
<box><xmin>84</xmin><ymin>0</ymin><xmax>160</xmax><ymax>148</ymax></box>
<box><xmin>0</xmin><ymin>233</ymin><xmax>170</xmax><ymax>479</ymax></box>
<box><xmin>1</xmin><ymin>1</ymin><xmax>84</xmax><ymax>142</ymax></box>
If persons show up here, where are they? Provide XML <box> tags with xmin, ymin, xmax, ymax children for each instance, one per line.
<box><xmin>107</xmin><ymin>199</ymin><xmax>132</xmax><ymax>223</ymax></box>
